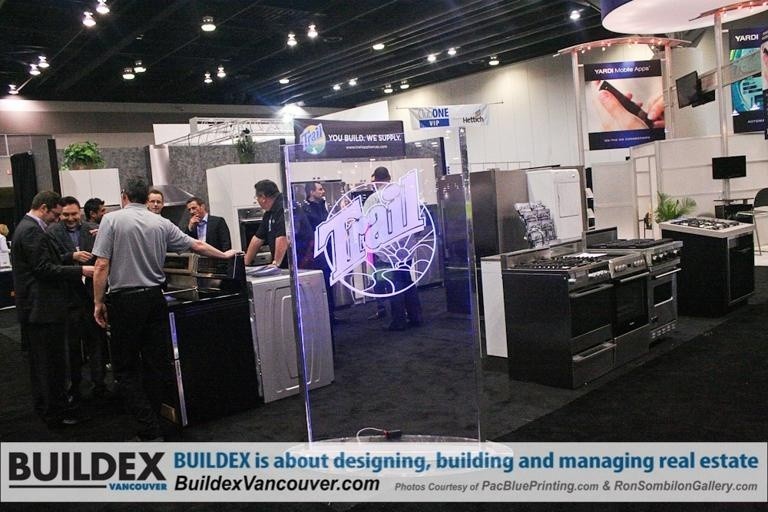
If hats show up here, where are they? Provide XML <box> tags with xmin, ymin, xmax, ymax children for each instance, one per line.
<box><xmin>370</xmin><ymin>166</ymin><xmax>391</xmax><ymax>181</ymax></box>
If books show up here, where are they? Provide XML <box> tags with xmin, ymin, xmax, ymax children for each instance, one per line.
<box><xmin>513</xmin><ymin>199</ymin><xmax>557</xmax><ymax>250</ymax></box>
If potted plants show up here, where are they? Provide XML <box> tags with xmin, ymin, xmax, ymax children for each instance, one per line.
<box><xmin>233</xmin><ymin>134</ymin><xmax>263</xmax><ymax>164</ymax></box>
<box><xmin>59</xmin><ymin>138</ymin><xmax>106</xmax><ymax>171</ymax></box>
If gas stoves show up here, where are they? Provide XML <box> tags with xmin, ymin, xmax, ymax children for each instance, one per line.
<box><xmin>672</xmin><ymin>214</ymin><xmax>742</xmax><ymax>231</ymax></box>
<box><xmin>164</xmin><ymin>287</ymin><xmax>238</xmax><ymax>306</ymax></box>
<box><xmin>507</xmin><ymin>236</ymin><xmax>685</xmax><ymax>294</ymax></box>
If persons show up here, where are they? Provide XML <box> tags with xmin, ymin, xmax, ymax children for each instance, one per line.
<box><xmin>359</xmin><ymin>166</ymin><xmax>424</xmax><ymax>332</ymax></box>
<box><xmin>8</xmin><ymin>188</ymin><xmax>232</xmax><ymax>428</ymax></box>
<box><xmin>0</xmin><ymin>222</ymin><xmax>12</xmax><ymax>254</ymax></box>
<box><xmin>762</xmin><ymin>48</ymin><xmax>768</xmax><ymax>83</ymax></box>
<box><xmin>588</xmin><ymin>77</ymin><xmax>664</xmax><ymax>131</ymax></box>
<box><xmin>242</xmin><ymin>178</ymin><xmax>316</xmax><ymax>270</ymax></box>
<box><xmin>297</xmin><ymin>180</ymin><xmax>336</xmax><ymax>358</ymax></box>
<box><xmin>365</xmin><ymin>251</ymin><xmax>394</xmax><ymax>322</ymax></box>
<box><xmin>89</xmin><ymin>174</ymin><xmax>247</xmax><ymax>441</ymax></box>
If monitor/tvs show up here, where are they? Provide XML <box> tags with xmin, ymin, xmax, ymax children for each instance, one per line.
<box><xmin>350</xmin><ymin>191</ymin><xmax>373</xmax><ymax>205</ymax></box>
<box><xmin>675</xmin><ymin>70</ymin><xmax>701</xmax><ymax>109</ymax></box>
<box><xmin>712</xmin><ymin>155</ymin><xmax>746</xmax><ymax>180</ymax></box>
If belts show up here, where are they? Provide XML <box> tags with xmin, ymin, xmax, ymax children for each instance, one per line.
<box><xmin>109</xmin><ymin>285</ymin><xmax>155</xmax><ymax>297</ymax></box>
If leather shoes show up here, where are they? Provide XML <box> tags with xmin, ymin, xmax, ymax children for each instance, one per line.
<box><xmin>34</xmin><ymin>393</ymin><xmax>93</xmax><ymax>431</ymax></box>
<box><xmin>365</xmin><ymin>310</ymin><xmax>424</xmax><ymax>332</ymax></box>
<box><xmin>122</xmin><ymin>431</ymin><xmax>170</xmax><ymax>442</ymax></box>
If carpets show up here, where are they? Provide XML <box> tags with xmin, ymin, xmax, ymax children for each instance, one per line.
<box><xmin>3</xmin><ymin>287</ymin><xmax>759</xmax><ymax>441</ymax></box>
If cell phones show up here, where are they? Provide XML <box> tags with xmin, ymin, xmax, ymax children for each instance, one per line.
<box><xmin>599</xmin><ymin>79</ymin><xmax>655</xmax><ymax>130</ymax></box>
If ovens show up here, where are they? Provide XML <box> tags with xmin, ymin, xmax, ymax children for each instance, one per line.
<box><xmin>554</xmin><ymin>261</ymin><xmax>680</xmax><ymax>388</ymax></box>
<box><xmin>160</xmin><ymin>300</ymin><xmax>260</xmax><ymax>428</ymax></box>
<box><xmin>239</xmin><ymin>218</ymin><xmax>273</xmax><ymax>266</ymax></box>
<box><xmin>729</xmin><ymin>245</ymin><xmax>755</xmax><ymax>304</ymax></box>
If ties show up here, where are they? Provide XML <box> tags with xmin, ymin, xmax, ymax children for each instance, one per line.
<box><xmin>196</xmin><ymin>221</ymin><xmax>206</xmax><ymax>241</ymax></box>
